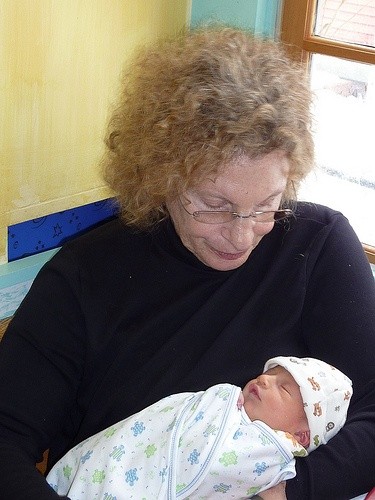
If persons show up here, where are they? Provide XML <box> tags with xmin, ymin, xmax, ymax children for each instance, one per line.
<box><xmin>0</xmin><ymin>21</ymin><xmax>375</xmax><ymax>500</ymax></box>
<box><xmin>44</xmin><ymin>356</ymin><xmax>354</xmax><ymax>500</ymax></box>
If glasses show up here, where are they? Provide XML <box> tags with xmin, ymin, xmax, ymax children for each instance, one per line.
<box><xmin>177</xmin><ymin>181</ymin><xmax>298</xmax><ymax>224</ymax></box>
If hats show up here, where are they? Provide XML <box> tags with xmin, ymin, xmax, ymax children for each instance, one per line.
<box><xmin>265</xmin><ymin>356</ymin><xmax>354</xmax><ymax>453</ymax></box>
